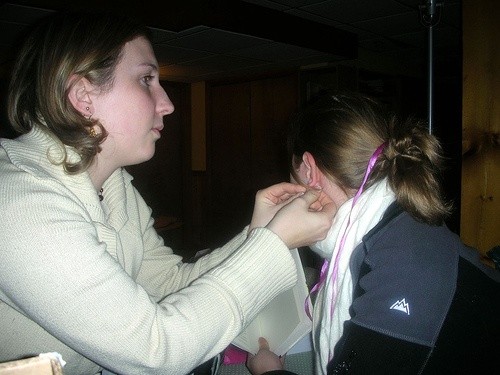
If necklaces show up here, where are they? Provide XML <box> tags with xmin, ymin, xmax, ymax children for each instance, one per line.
<box><xmin>98</xmin><ymin>187</ymin><xmax>104</xmax><ymax>202</ymax></box>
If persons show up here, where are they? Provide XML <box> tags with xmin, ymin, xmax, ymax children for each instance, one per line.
<box><xmin>0</xmin><ymin>7</ymin><xmax>337</xmax><ymax>375</ymax></box>
<box><xmin>246</xmin><ymin>91</ymin><xmax>500</xmax><ymax>375</ymax></box>
